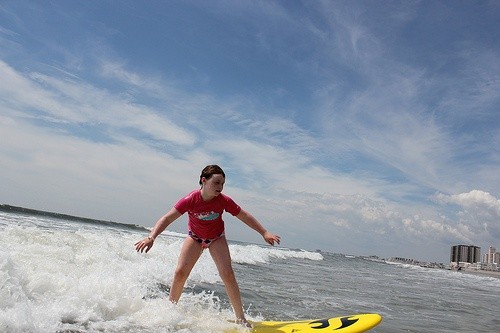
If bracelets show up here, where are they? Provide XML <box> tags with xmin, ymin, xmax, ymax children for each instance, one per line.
<box><xmin>148</xmin><ymin>234</ymin><xmax>157</xmax><ymax>244</ymax></box>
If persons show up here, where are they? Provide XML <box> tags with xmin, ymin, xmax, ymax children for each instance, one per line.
<box><xmin>137</xmin><ymin>164</ymin><xmax>282</xmax><ymax>328</ymax></box>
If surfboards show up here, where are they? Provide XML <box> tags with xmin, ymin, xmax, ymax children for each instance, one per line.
<box><xmin>174</xmin><ymin>311</ymin><xmax>383</xmax><ymax>333</ymax></box>
